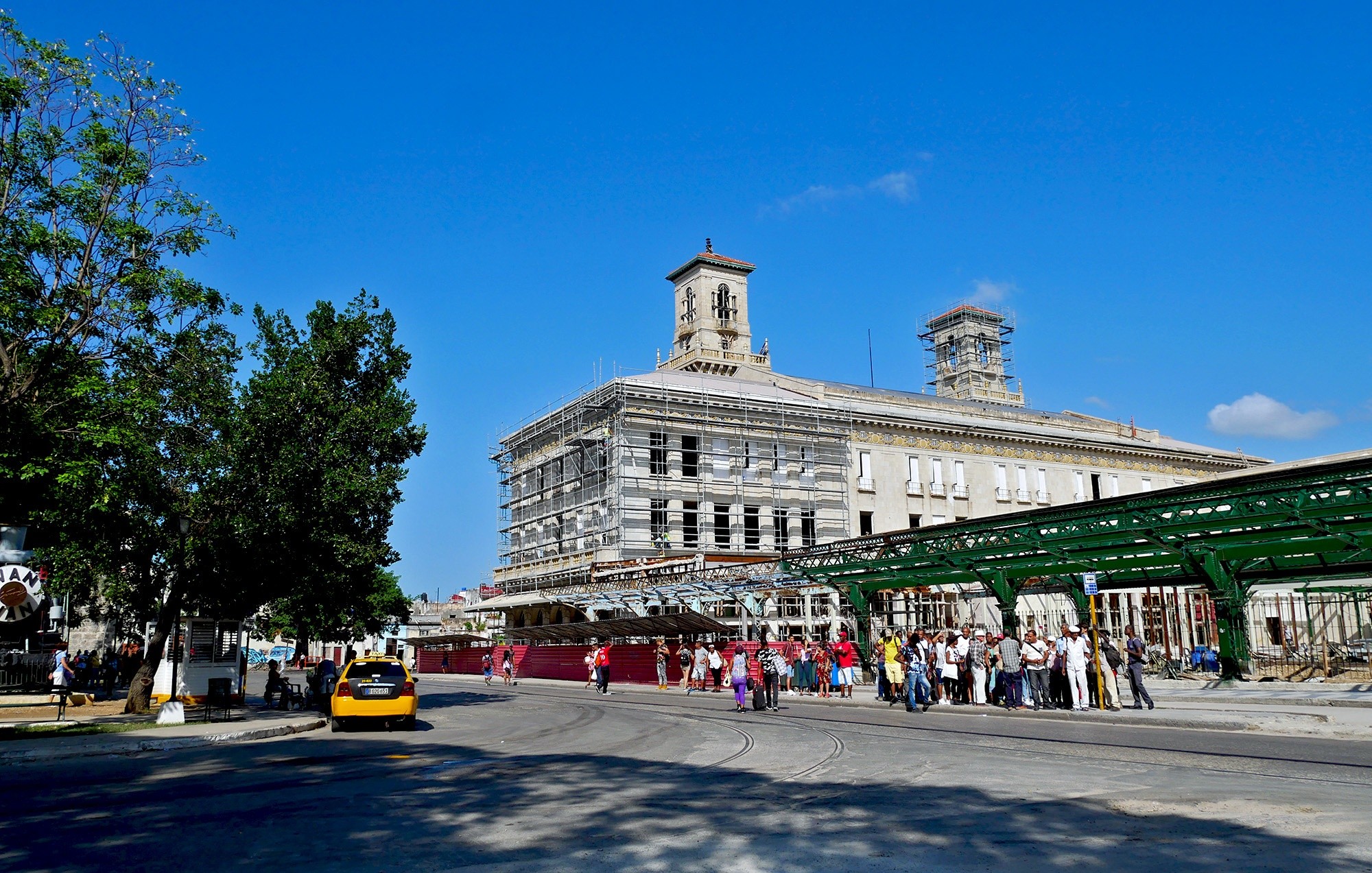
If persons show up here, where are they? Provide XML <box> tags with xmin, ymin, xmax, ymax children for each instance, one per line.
<box><xmin>279</xmin><ymin>653</ymin><xmax>287</xmax><ymax>674</ymax></box>
<box><xmin>441</xmin><ymin>651</ymin><xmax>449</xmax><ymax>674</ymax></box>
<box><xmin>318</xmin><ymin>660</ymin><xmax>336</xmax><ymax>723</ymax></box>
<box><xmin>754</xmin><ymin>638</ymin><xmax>773</xmax><ymax>691</ymax></box>
<box><xmin>875</xmin><ymin>618</ymin><xmax>1122</xmax><ymax>713</ymax></box>
<box><xmin>796</xmin><ymin>639</ymin><xmax>814</xmax><ymax>696</ymax></box>
<box><xmin>780</xmin><ymin>634</ymin><xmax>797</xmax><ymax>696</ymax></box>
<box><xmin>585</xmin><ymin>640</ymin><xmax>613</xmax><ymax>695</ymax></box>
<box><xmin>1122</xmin><ymin>624</ymin><xmax>1154</xmax><ymax>710</ymax></box>
<box><xmin>410</xmin><ymin>657</ymin><xmax>416</xmax><ymax>673</ymax></box>
<box><xmin>266</xmin><ymin>659</ymin><xmax>290</xmax><ymax>710</ymax></box>
<box><xmin>812</xmin><ymin>640</ymin><xmax>836</xmax><ymax>698</ymax></box>
<box><xmin>653</xmin><ymin>638</ymin><xmax>670</xmax><ymax>690</ymax></box>
<box><xmin>222</xmin><ymin>643</ymin><xmax>246</xmax><ymax>697</ymax></box>
<box><xmin>1284</xmin><ymin>625</ymin><xmax>1293</xmax><ymax>654</ymax></box>
<box><xmin>481</xmin><ymin>650</ymin><xmax>496</xmax><ymax>686</ymax></box>
<box><xmin>757</xmin><ymin>640</ymin><xmax>783</xmax><ymax>711</ymax></box>
<box><xmin>833</xmin><ymin>632</ymin><xmax>853</xmax><ymax>700</ymax></box>
<box><xmin>502</xmin><ymin>650</ymin><xmax>512</xmax><ymax>686</ymax></box>
<box><xmin>346</xmin><ymin>650</ymin><xmax>357</xmax><ymax>666</ymax></box>
<box><xmin>691</xmin><ymin>641</ymin><xmax>709</xmax><ymax>691</ymax></box>
<box><xmin>48</xmin><ymin>641</ymin><xmax>74</xmax><ymax>703</ymax></box>
<box><xmin>733</xmin><ymin>643</ymin><xmax>750</xmax><ymax>694</ymax></box>
<box><xmin>676</xmin><ymin>640</ymin><xmax>694</xmax><ymax>689</ymax></box>
<box><xmin>728</xmin><ymin>645</ymin><xmax>751</xmax><ymax>713</ymax></box>
<box><xmin>706</xmin><ymin>645</ymin><xmax>724</xmax><ymax>692</ymax></box>
<box><xmin>298</xmin><ymin>652</ymin><xmax>306</xmax><ymax>670</ymax></box>
<box><xmin>64</xmin><ymin>647</ymin><xmax>143</xmax><ymax>696</ymax></box>
<box><xmin>504</xmin><ymin>643</ymin><xmax>516</xmax><ymax>678</ymax></box>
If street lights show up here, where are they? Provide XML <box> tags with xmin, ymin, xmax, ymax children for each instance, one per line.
<box><xmin>165</xmin><ymin>514</ymin><xmax>191</xmax><ymax>702</ymax></box>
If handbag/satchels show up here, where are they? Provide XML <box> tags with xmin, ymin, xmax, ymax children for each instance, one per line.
<box><xmin>1103</xmin><ymin>643</ymin><xmax>1125</xmax><ymax>674</ymax></box>
<box><xmin>723</xmin><ymin>667</ymin><xmax>731</xmax><ymax>687</ymax></box>
<box><xmin>984</xmin><ymin>657</ymin><xmax>996</xmax><ymax>669</ymax></box>
<box><xmin>680</xmin><ymin>653</ymin><xmax>689</xmax><ymax>665</ymax></box>
<box><xmin>412</xmin><ymin>664</ymin><xmax>415</xmax><ymax>667</ymax></box>
<box><xmin>1044</xmin><ymin>659</ymin><xmax>1046</xmax><ymax>665</ymax></box>
<box><xmin>924</xmin><ymin>649</ymin><xmax>930</xmax><ymax>659</ymax></box>
<box><xmin>773</xmin><ymin>649</ymin><xmax>787</xmax><ymax>676</ymax></box>
<box><xmin>746</xmin><ymin>675</ymin><xmax>754</xmax><ymax>691</ymax></box>
<box><xmin>986</xmin><ymin>670</ymin><xmax>990</xmax><ymax>682</ymax></box>
<box><xmin>723</xmin><ymin>658</ymin><xmax>728</xmax><ymax>667</ymax></box>
<box><xmin>959</xmin><ymin>655</ymin><xmax>967</xmax><ymax>671</ymax></box>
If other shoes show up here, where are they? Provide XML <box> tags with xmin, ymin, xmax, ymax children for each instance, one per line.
<box><xmin>786</xmin><ymin>689</ymin><xmax>853</xmax><ymax>700</ymax></box>
<box><xmin>876</xmin><ymin>692</ymin><xmax>1123</xmax><ymax>713</ymax></box>
<box><xmin>682</xmin><ymin>686</ymin><xmax>720</xmax><ymax>693</ymax></box>
<box><xmin>596</xmin><ymin>687</ymin><xmax>600</xmax><ymax>693</ymax></box>
<box><xmin>657</xmin><ymin>683</ymin><xmax>667</xmax><ymax>690</ymax></box>
<box><xmin>765</xmin><ymin>705</ymin><xmax>779</xmax><ymax>712</ymax></box>
<box><xmin>1147</xmin><ymin>700</ymin><xmax>1154</xmax><ymax>709</ymax></box>
<box><xmin>602</xmin><ymin>692</ymin><xmax>611</xmax><ymax>695</ymax></box>
<box><xmin>1130</xmin><ymin>705</ymin><xmax>1142</xmax><ymax>709</ymax></box>
<box><xmin>736</xmin><ymin>706</ymin><xmax>746</xmax><ymax>714</ymax></box>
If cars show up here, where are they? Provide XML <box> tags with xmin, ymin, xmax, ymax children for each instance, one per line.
<box><xmin>329</xmin><ymin>651</ymin><xmax>419</xmax><ymax>733</ymax></box>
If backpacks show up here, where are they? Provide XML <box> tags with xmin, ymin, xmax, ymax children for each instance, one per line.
<box><xmin>48</xmin><ymin>655</ymin><xmax>58</xmax><ymax>673</ymax></box>
<box><xmin>1133</xmin><ymin>637</ymin><xmax>1151</xmax><ymax>664</ymax></box>
<box><xmin>1049</xmin><ymin>650</ymin><xmax>1061</xmax><ymax>671</ymax></box>
<box><xmin>483</xmin><ymin>659</ymin><xmax>490</xmax><ymax>668</ymax></box>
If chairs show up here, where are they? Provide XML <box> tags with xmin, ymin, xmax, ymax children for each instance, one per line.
<box><xmin>204</xmin><ymin>677</ymin><xmax>231</xmax><ymax>723</ymax></box>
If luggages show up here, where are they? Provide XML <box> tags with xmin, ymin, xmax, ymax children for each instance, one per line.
<box><xmin>752</xmin><ymin>686</ymin><xmax>766</xmax><ymax>709</ymax></box>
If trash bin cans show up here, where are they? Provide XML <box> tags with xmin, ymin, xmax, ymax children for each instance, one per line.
<box><xmin>779</xmin><ymin>658</ymin><xmax>854</xmax><ymax>691</ymax></box>
<box><xmin>1191</xmin><ymin>646</ymin><xmax>1220</xmax><ymax>673</ymax></box>
<box><xmin>206</xmin><ymin>678</ymin><xmax>234</xmax><ymax>709</ymax></box>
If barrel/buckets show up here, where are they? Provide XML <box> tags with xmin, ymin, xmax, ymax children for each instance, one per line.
<box><xmin>1203</xmin><ymin>651</ymin><xmax>1219</xmax><ymax>672</ymax></box>
<box><xmin>1191</xmin><ymin>651</ymin><xmax>1205</xmax><ymax>672</ymax></box>
<box><xmin>1195</xmin><ymin>646</ymin><xmax>1208</xmax><ymax>651</ymax></box>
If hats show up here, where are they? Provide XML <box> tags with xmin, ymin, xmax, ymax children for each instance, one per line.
<box><xmin>1069</xmin><ymin>625</ymin><xmax>1080</xmax><ymax>633</ymax></box>
<box><xmin>1047</xmin><ymin>636</ymin><xmax>1056</xmax><ymax>641</ymax></box>
<box><xmin>997</xmin><ymin>634</ymin><xmax>1004</xmax><ymax>639</ymax></box>
<box><xmin>85</xmin><ymin>650</ymin><xmax>89</xmax><ymax>654</ymax></box>
<box><xmin>975</xmin><ymin>630</ymin><xmax>986</xmax><ymax>636</ymax></box>
<box><xmin>945</xmin><ymin>635</ymin><xmax>962</xmax><ymax>647</ymax></box>
<box><xmin>884</xmin><ymin>629</ymin><xmax>892</xmax><ymax>637</ymax></box>
<box><xmin>838</xmin><ymin>632</ymin><xmax>847</xmax><ymax>636</ymax></box>
